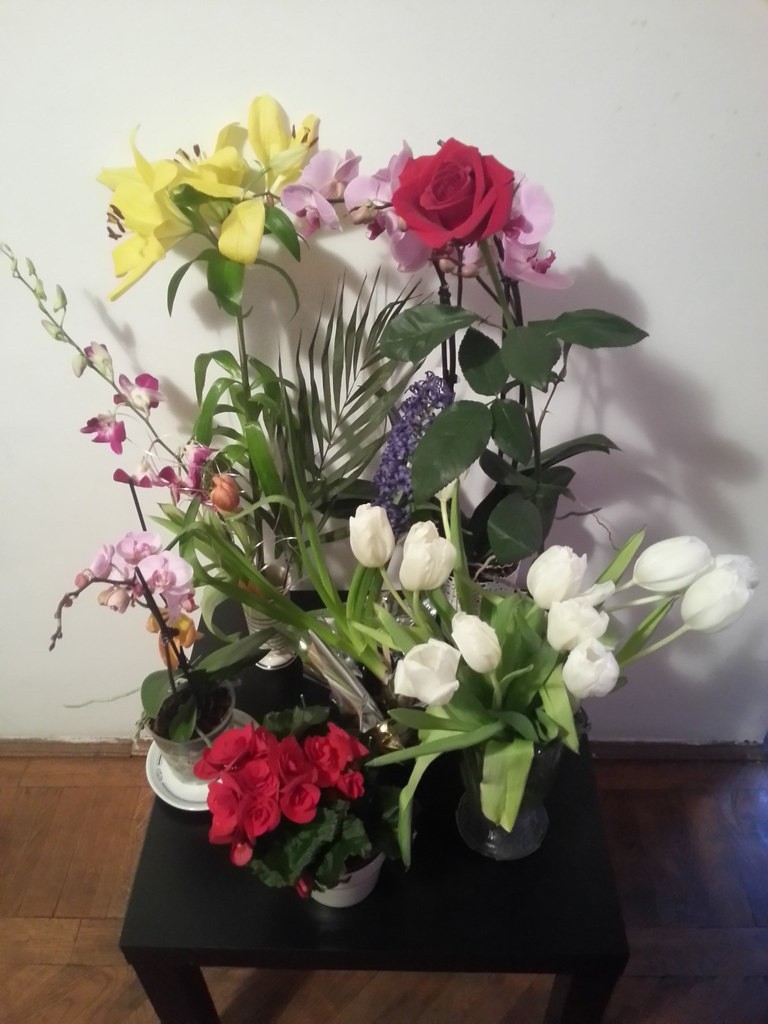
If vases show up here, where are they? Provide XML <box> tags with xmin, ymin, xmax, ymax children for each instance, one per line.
<box><xmin>311</xmin><ymin>853</ymin><xmax>383</xmax><ymax>909</ymax></box>
<box><xmin>455</xmin><ymin>733</ymin><xmax>549</xmax><ymax>859</ymax></box>
<box><xmin>241</xmin><ymin>565</ymin><xmax>297</xmax><ymax>671</ymax></box>
<box><xmin>133</xmin><ymin>681</ymin><xmax>259</xmax><ymax>814</ymax></box>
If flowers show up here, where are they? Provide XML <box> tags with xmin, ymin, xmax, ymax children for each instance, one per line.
<box><xmin>2</xmin><ymin>91</ymin><xmax>762</xmax><ymax>897</ymax></box>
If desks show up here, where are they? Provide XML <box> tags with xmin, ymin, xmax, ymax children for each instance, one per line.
<box><xmin>118</xmin><ymin>724</ymin><xmax>630</xmax><ymax>1024</ymax></box>
